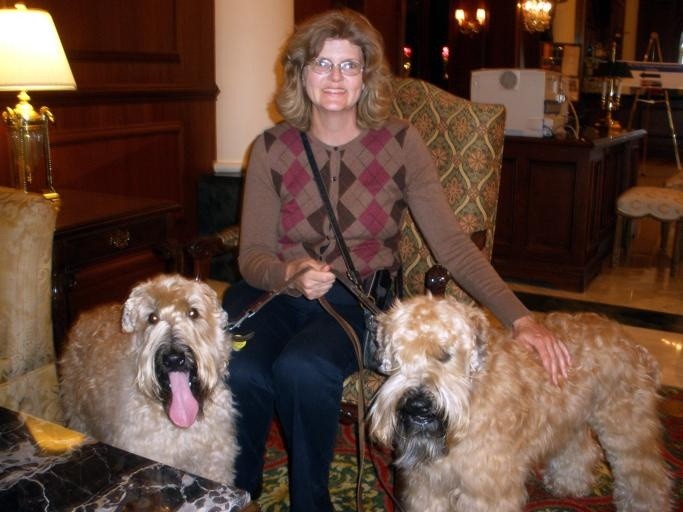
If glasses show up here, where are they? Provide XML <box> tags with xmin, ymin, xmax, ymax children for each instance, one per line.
<box><xmin>307</xmin><ymin>56</ymin><xmax>363</xmax><ymax>77</ymax></box>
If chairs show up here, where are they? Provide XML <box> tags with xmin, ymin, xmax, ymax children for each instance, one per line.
<box><xmin>1</xmin><ymin>184</ymin><xmax>62</xmax><ymax>385</ymax></box>
<box><xmin>269</xmin><ymin>72</ymin><xmax>509</xmax><ymax>508</ymax></box>
<box><xmin>186</xmin><ymin>171</ymin><xmax>244</xmax><ymax>278</ymax></box>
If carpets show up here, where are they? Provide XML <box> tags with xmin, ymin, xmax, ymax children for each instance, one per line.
<box><xmin>256</xmin><ymin>385</ymin><xmax>683</xmax><ymax>511</ymax></box>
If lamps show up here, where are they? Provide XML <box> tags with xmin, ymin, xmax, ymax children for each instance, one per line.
<box><xmin>453</xmin><ymin>1</ymin><xmax>490</xmax><ymax>39</ymax></box>
<box><xmin>516</xmin><ymin>0</ymin><xmax>555</xmax><ymax>35</ymax></box>
<box><xmin>0</xmin><ymin>3</ymin><xmax>82</xmax><ymax>201</ymax></box>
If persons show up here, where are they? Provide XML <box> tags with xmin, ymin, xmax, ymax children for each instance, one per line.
<box><xmin>221</xmin><ymin>10</ymin><xmax>575</xmax><ymax>512</ymax></box>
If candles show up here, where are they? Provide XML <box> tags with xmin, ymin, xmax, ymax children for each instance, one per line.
<box><xmin>600</xmin><ymin>77</ymin><xmax>623</xmax><ymax>100</ymax></box>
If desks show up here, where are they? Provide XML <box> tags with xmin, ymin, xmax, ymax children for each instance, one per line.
<box><xmin>0</xmin><ymin>405</ymin><xmax>256</xmax><ymax>511</ymax></box>
<box><xmin>37</xmin><ymin>186</ymin><xmax>184</xmax><ymax>322</ymax></box>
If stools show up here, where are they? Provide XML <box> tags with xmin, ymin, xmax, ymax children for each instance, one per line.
<box><xmin>612</xmin><ymin>184</ymin><xmax>683</xmax><ymax>277</ymax></box>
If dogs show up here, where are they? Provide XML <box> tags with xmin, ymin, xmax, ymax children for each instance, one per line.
<box><xmin>49</xmin><ymin>272</ymin><xmax>243</xmax><ymax>489</ymax></box>
<box><xmin>364</xmin><ymin>289</ymin><xmax>682</xmax><ymax>512</ymax></box>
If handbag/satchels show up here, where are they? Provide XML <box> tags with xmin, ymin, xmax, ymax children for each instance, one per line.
<box><xmin>355</xmin><ymin>263</ymin><xmax>405</xmax><ymax>372</ymax></box>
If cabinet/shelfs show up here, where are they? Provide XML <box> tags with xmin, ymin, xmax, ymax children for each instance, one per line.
<box><xmin>1</xmin><ymin>3</ymin><xmax>225</xmax><ymax>313</ymax></box>
